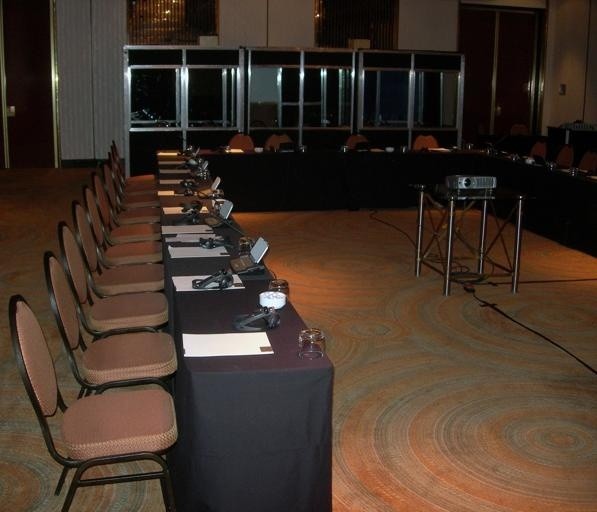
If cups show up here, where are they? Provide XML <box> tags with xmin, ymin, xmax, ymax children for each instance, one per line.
<box><xmin>296</xmin><ymin>328</ymin><xmax>326</xmax><ymax>361</ymax></box>
<box><xmin>238</xmin><ymin>237</ymin><xmax>254</xmax><ymax>257</ymax></box>
<box><xmin>268</xmin><ymin>278</ymin><xmax>289</xmax><ymax>306</ymax></box>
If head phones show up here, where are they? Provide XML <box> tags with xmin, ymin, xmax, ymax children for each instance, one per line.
<box><xmin>199</xmin><ymin>236</ymin><xmax>235</xmax><ymax>249</ymax></box>
<box><xmin>232</xmin><ymin>306</ymin><xmax>281</xmax><ymax>329</ymax></box>
<box><xmin>192</xmin><ymin>269</ymin><xmax>234</xmax><ymax>289</ymax></box>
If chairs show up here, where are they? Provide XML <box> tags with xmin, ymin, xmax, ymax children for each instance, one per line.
<box><xmin>59</xmin><ymin>138</ymin><xmax>169</xmax><ymax>397</ymax></box>
<box><xmin>10</xmin><ymin>294</ymin><xmax>177</xmax><ymax>512</ymax></box>
<box><xmin>44</xmin><ymin>252</ymin><xmax>178</xmax><ymax>494</ymax></box>
<box><xmin>527</xmin><ymin>142</ymin><xmax>546</xmax><ymax>162</ymax></box>
<box><xmin>412</xmin><ymin>135</ymin><xmax>438</xmax><ymax>151</ymax></box>
<box><xmin>226</xmin><ymin>134</ymin><xmax>291</xmax><ymax>151</ymax></box>
<box><xmin>343</xmin><ymin>134</ymin><xmax>367</xmax><ymax>150</ymax></box>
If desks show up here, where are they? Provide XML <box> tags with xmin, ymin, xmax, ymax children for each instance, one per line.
<box><xmin>169</xmin><ymin>280</ymin><xmax>334</xmax><ymax>512</ymax></box>
<box><xmin>465</xmin><ymin>149</ymin><xmax>596</xmax><ymax>256</ymax></box>
<box><xmin>159</xmin><ymin>150</ymin><xmax>274</xmax><ymax>287</ymax></box>
<box><xmin>203</xmin><ymin>147</ymin><xmax>492</xmax><ymax>212</ymax></box>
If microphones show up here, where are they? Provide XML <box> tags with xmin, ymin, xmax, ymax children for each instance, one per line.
<box><xmin>175</xmin><ymin>134</ymin><xmax>247</xmax><ymax>237</ymax></box>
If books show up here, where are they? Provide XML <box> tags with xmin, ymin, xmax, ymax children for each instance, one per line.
<box><xmin>156</xmin><ymin>152</ymin><xmax>178</xmax><ymax>156</ymax></box>
<box><xmin>159</xmin><ymin>179</ymin><xmax>196</xmax><ymax>185</ymax></box>
<box><xmin>163</xmin><ymin>205</ymin><xmax>210</xmax><ymax>215</ymax></box>
<box><xmin>162</xmin><ymin>224</ymin><xmax>214</xmax><ymax>235</ymax></box>
<box><xmin>171</xmin><ymin>273</ymin><xmax>245</xmax><ymax>294</ymax></box>
<box><xmin>229</xmin><ymin>148</ymin><xmax>244</xmax><ymax>153</ymax></box>
<box><xmin>158</xmin><ymin>160</ymin><xmax>186</xmax><ymax>165</ymax></box>
<box><xmin>168</xmin><ymin>244</ymin><xmax>231</xmax><ymax>261</ymax></box>
<box><xmin>158</xmin><ymin>191</ymin><xmax>175</xmax><ymax>196</ymax></box>
<box><xmin>182</xmin><ymin>331</ymin><xmax>275</xmax><ymax>358</ymax></box>
<box><xmin>159</xmin><ymin>168</ymin><xmax>192</xmax><ymax>174</ymax></box>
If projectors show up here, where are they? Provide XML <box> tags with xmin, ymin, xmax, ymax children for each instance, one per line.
<box><xmin>445</xmin><ymin>175</ymin><xmax>497</xmax><ymax>189</ymax></box>
